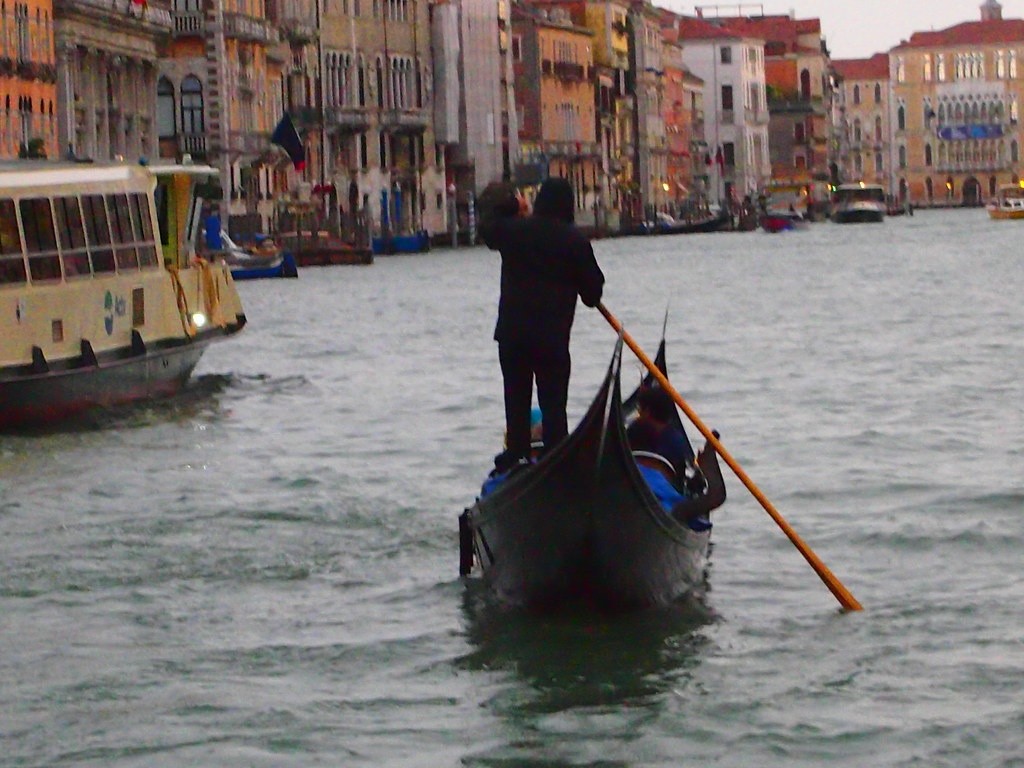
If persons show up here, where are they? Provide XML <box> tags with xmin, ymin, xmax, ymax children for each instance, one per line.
<box><xmin>626</xmin><ymin>385</ymin><xmax>687</xmax><ymax>495</ymax></box>
<box><xmin>480</xmin><ymin>176</ymin><xmax>605</xmax><ymax>473</ymax></box>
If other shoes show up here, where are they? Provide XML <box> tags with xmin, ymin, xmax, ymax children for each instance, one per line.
<box><xmin>492</xmin><ymin>450</ymin><xmax>533</xmax><ymax>471</ymax></box>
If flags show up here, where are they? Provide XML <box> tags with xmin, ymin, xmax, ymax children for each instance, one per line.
<box><xmin>270</xmin><ymin>111</ymin><xmax>306</xmax><ymax>172</ymax></box>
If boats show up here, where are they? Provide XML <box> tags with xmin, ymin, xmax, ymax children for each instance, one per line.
<box><xmin>457</xmin><ymin>308</ymin><xmax>726</xmax><ymax>618</ymax></box>
<box><xmin>651</xmin><ymin>194</ymin><xmax>792</xmax><ymax>236</ymax></box>
<box><xmin>828</xmin><ymin>182</ymin><xmax>887</xmax><ymax>223</ymax></box>
<box><xmin>222</xmin><ymin>229</ymin><xmax>432</xmax><ymax>281</ymax></box>
<box><xmin>985</xmin><ymin>183</ymin><xmax>1024</xmax><ymax>221</ymax></box>
<box><xmin>0</xmin><ymin>153</ymin><xmax>248</xmax><ymax>427</ymax></box>
